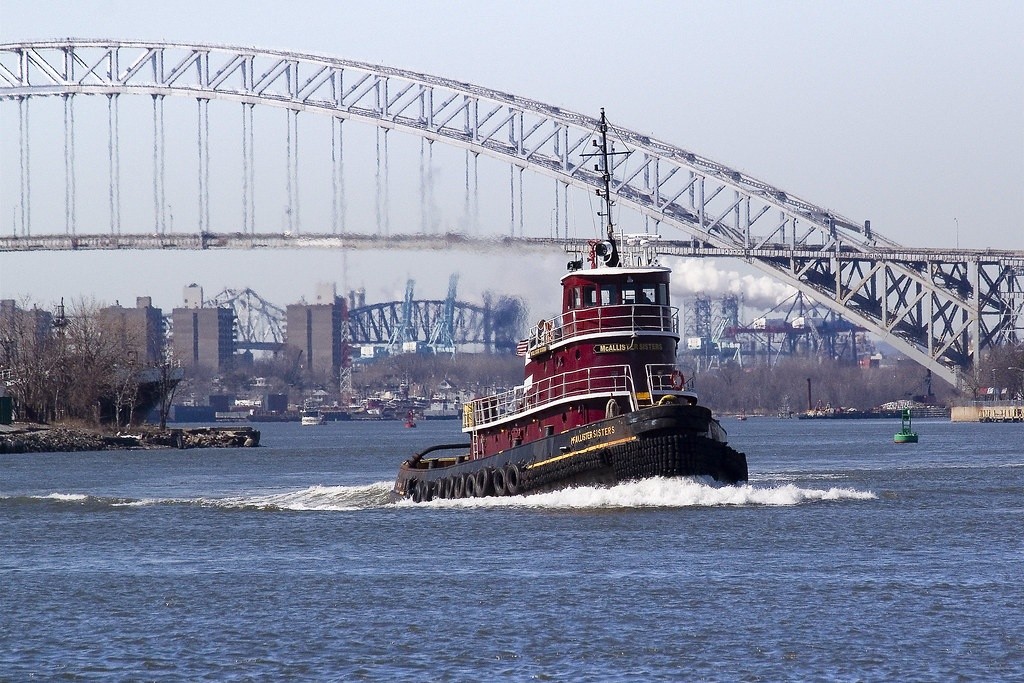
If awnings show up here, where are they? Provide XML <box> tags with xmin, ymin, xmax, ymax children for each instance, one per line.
<box><xmin>979</xmin><ymin>387</ymin><xmax>1012</xmax><ymax>396</ymax></box>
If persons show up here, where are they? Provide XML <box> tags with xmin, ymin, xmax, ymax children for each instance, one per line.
<box><xmin>643</xmin><ymin>291</ymin><xmax>650</xmax><ymax>302</ymax></box>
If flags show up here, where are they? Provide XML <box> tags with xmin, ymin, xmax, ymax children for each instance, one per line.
<box><xmin>516</xmin><ymin>338</ymin><xmax>528</xmax><ymax>356</ymax></box>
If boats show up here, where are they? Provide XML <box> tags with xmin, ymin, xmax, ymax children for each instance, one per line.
<box><xmin>405</xmin><ymin>411</ymin><xmax>415</xmax><ymax>428</ymax></box>
<box><xmin>303</xmin><ymin>412</ymin><xmax>324</xmax><ymax>427</ymax></box>
<box><xmin>392</xmin><ymin>109</ymin><xmax>751</xmax><ymax>504</ymax></box>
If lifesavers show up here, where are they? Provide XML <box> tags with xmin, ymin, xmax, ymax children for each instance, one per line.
<box><xmin>670</xmin><ymin>370</ymin><xmax>685</xmax><ymax>390</ymax></box>
